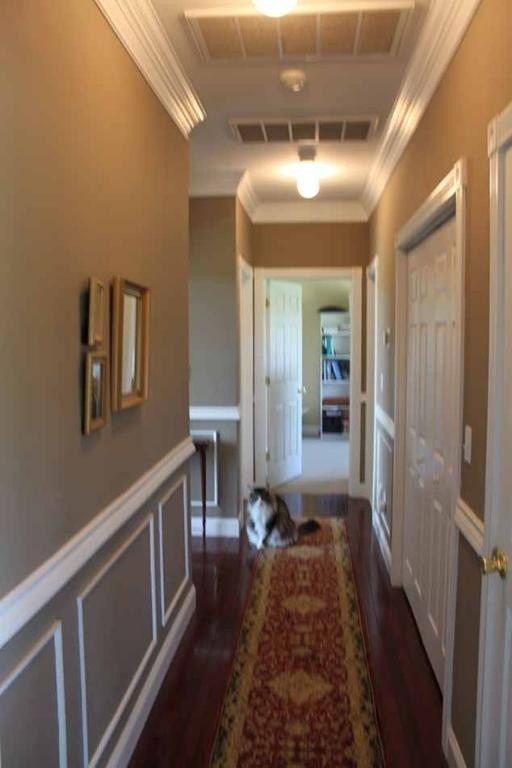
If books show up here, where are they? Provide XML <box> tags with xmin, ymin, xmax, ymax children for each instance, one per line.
<box><xmin>322</xmin><ymin>335</ymin><xmax>344</xmax><ymax>380</ymax></box>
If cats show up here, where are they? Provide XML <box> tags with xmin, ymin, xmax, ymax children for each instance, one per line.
<box><xmin>243</xmin><ymin>480</ymin><xmax>321</xmax><ymax>551</ymax></box>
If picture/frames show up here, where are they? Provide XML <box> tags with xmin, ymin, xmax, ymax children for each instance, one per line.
<box><xmin>82</xmin><ymin>273</ymin><xmax>152</xmax><ymax>438</ymax></box>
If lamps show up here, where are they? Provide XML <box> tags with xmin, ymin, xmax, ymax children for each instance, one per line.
<box><xmin>280</xmin><ymin>145</ymin><xmax>337</xmax><ymax>199</ymax></box>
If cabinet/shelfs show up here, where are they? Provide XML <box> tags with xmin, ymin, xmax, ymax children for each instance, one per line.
<box><xmin>318</xmin><ymin>311</ymin><xmax>349</xmax><ymax>442</ymax></box>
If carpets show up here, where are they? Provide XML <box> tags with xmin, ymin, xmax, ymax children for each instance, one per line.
<box><xmin>209</xmin><ymin>513</ymin><xmax>385</xmax><ymax>768</ymax></box>
